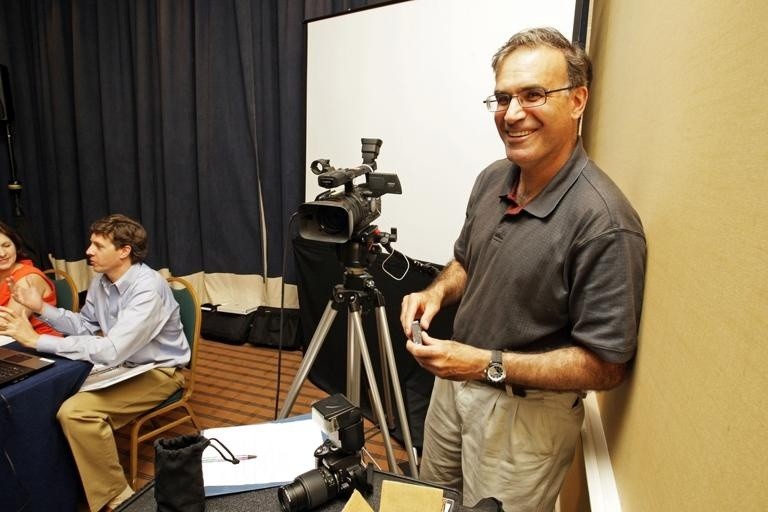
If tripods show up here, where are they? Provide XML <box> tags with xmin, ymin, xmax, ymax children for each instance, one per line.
<box><xmin>276</xmin><ymin>268</ymin><xmax>420</xmax><ymax>481</ymax></box>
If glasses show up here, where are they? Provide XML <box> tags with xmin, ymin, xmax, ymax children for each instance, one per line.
<box><xmin>483</xmin><ymin>88</ymin><xmax>576</xmax><ymax>112</ymax></box>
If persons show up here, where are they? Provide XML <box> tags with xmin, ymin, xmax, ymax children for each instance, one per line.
<box><xmin>0</xmin><ymin>214</ymin><xmax>191</xmax><ymax>512</ymax></box>
<box><xmin>0</xmin><ymin>221</ymin><xmax>64</xmax><ymax>337</ymax></box>
<box><xmin>400</xmin><ymin>26</ymin><xmax>647</xmax><ymax>512</ymax></box>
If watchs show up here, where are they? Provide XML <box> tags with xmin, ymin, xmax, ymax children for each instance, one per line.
<box><xmin>484</xmin><ymin>350</ymin><xmax>506</xmax><ymax>388</ymax></box>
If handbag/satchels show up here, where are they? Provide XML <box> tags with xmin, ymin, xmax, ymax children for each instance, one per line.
<box><xmin>200</xmin><ymin>312</ymin><xmax>251</xmax><ymax>344</ymax></box>
<box><xmin>249</xmin><ymin>309</ymin><xmax>302</xmax><ymax>351</ymax></box>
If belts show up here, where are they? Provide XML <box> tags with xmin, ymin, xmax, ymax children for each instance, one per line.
<box><xmin>473</xmin><ymin>379</ymin><xmax>526</xmax><ymax>398</ymax></box>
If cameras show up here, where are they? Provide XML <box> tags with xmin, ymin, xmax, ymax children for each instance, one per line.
<box><xmin>277</xmin><ymin>393</ymin><xmax>374</xmax><ymax>512</ymax></box>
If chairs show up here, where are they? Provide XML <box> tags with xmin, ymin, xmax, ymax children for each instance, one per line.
<box><xmin>130</xmin><ymin>277</ymin><xmax>202</xmax><ymax>491</ymax></box>
<box><xmin>43</xmin><ymin>269</ymin><xmax>79</xmax><ymax>312</ymax></box>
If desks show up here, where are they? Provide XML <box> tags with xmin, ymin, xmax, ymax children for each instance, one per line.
<box><xmin>111</xmin><ymin>413</ymin><xmax>447</xmax><ymax>512</ymax></box>
<box><xmin>0</xmin><ymin>340</ymin><xmax>94</xmax><ymax>512</ymax></box>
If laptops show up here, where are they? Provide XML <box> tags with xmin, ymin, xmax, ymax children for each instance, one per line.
<box><xmin>0</xmin><ymin>347</ymin><xmax>57</xmax><ymax>389</ymax></box>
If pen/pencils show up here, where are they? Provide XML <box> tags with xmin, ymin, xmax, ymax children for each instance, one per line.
<box><xmin>201</xmin><ymin>454</ymin><xmax>257</xmax><ymax>463</ymax></box>
